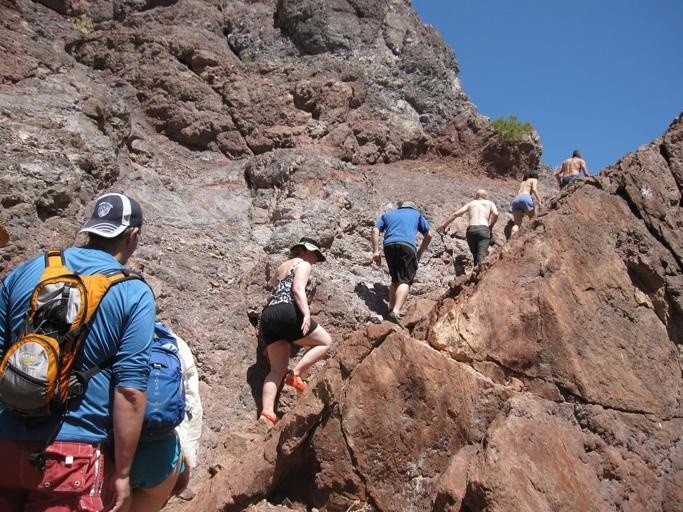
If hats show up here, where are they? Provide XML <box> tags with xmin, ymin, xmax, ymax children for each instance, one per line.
<box><xmin>398</xmin><ymin>201</ymin><xmax>417</xmax><ymax>210</ymax></box>
<box><xmin>290</xmin><ymin>237</ymin><xmax>327</xmax><ymax>262</ymax></box>
<box><xmin>79</xmin><ymin>194</ymin><xmax>142</xmax><ymax>238</ymax></box>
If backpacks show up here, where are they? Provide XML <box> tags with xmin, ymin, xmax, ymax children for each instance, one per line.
<box><xmin>0</xmin><ymin>250</ymin><xmax>145</xmax><ymax>418</ymax></box>
<box><xmin>144</xmin><ymin>324</ymin><xmax>186</xmax><ymax>432</ymax></box>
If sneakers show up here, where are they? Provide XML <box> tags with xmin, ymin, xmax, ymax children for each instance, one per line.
<box><xmin>260</xmin><ymin>411</ymin><xmax>279</xmax><ymax>423</ymax></box>
<box><xmin>285</xmin><ymin>370</ymin><xmax>304</xmax><ymax>391</ymax></box>
<box><xmin>387</xmin><ymin>309</ymin><xmax>404</xmax><ymax>328</ymax></box>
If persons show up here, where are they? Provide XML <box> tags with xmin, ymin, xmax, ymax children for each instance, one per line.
<box><xmin>1</xmin><ymin>193</ymin><xmax>154</xmax><ymax>512</ymax></box>
<box><xmin>259</xmin><ymin>235</ymin><xmax>331</xmax><ymax>423</ymax></box>
<box><xmin>511</xmin><ymin>170</ymin><xmax>542</xmax><ymax>234</ymax></box>
<box><xmin>372</xmin><ymin>202</ymin><xmax>432</xmax><ymax>324</ymax></box>
<box><xmin>107</xmin><ymin>329</ymin><xmax>200</xmax><ymax>512</ymax></box>
<box><xmin>555</xmin><ymin>151</ymin><xmax>590</xmax><ymax>190</ymax></box>
<box><xmin>436</xmin><ymin>188</ymin><xmax>499</xmax><ymax>268</ymax></box>
